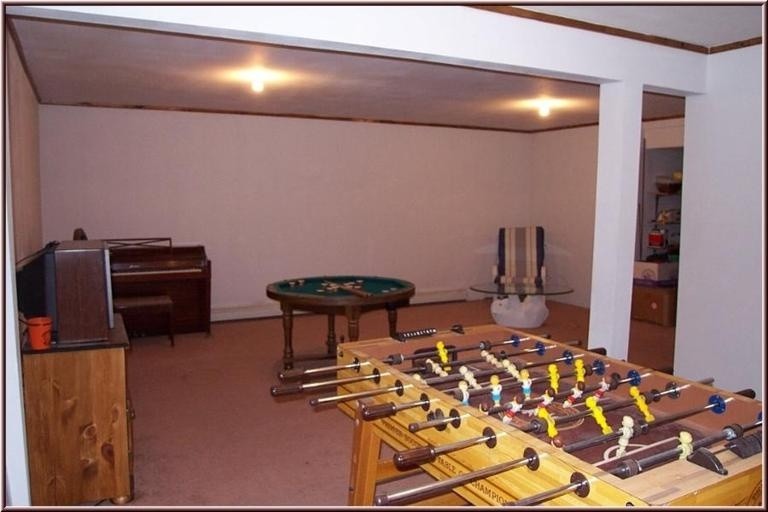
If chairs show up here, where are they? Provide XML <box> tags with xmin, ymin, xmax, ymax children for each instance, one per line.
<box><xmin>496</xmin><ymin>225</ymin><xmax>547</xmax><ymax>294</ymax></box>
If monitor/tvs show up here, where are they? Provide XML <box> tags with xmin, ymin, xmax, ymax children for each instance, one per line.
<box><xmin>17</xmin><ymin>239</ymin><xmax>114</xmax><ymax>344</ymax></box>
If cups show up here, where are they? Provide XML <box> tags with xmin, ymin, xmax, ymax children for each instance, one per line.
<box><xmin>27</xmin><ymin>317</ymin><xmax>53</xmax><ymax>350</ymax></box>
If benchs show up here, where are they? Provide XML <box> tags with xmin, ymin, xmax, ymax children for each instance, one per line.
<box><xmin>112</xmin><ymin>294</ymin><xmax>175</xmax><ymax>346</ymax></box>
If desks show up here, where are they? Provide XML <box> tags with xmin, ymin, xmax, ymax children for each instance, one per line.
<box><xmin>265</xmin><ymin>274</ymin><xmax>415</xmax><ymax>369</ymax></box>
<box><xmin>469</xmin><ymin>282</ymin><xmax>575</xmax><ymax>329</ymax></box>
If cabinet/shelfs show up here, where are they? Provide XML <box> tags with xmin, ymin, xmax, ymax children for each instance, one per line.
<box><xmin>21</xmin><ymin>310</ymin><xmax>136</xmax><ymax>506</ymax></box>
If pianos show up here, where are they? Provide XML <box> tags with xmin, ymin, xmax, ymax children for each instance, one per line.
<box><xmin>103</xmin><ymin>238</ymin><xmax>211</xmax><ymax>338</ymax></box>
